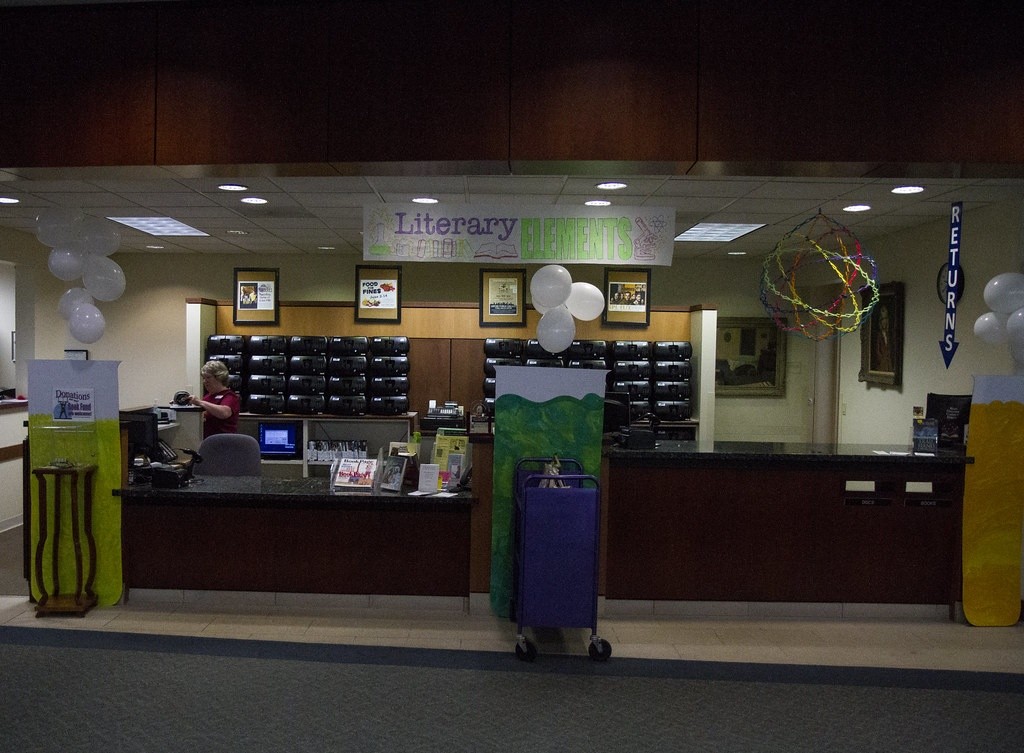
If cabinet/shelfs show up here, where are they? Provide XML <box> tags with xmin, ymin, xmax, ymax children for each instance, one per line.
<box><xmin>237</xmin><ymin>416</ymin><xmax>411</xmax><ymax>478</ymax></box>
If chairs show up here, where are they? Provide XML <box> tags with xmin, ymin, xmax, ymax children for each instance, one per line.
<box><xmin>193</xmin><ymin>433</ymin><xmax>261</xmax><ymax>477</ymax></box>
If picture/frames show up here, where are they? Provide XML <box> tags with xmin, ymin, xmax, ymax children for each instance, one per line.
<box><xmin>603</xmin><ymin>267</ymin><xmax>652</xmax><ymax>326</ymax></box>
<box><xmin>858</xmin><ymin>281</ymin><xmax>905</xmax><ymax>385</ymax></box>
<box><xmin>715</xmin><ymin>317</ymin><xmax>787</xmax><ymax>398</ymax></box>
<box><xmin>355</xmin><ymin>265</ymin><xmax>402</xmax><ymax>323</ymax></box>
<box><xmin>233</xmin><ymin>268</ymin><xmax>279</xmax><ymax>326</ymax></box>
<box><xmin>479</xmin><ymin>268</ymin><xmax>526</xmax><ymax>327</ymax></box>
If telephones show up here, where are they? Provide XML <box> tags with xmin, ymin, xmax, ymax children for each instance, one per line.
<box><xmin>457</xmin><ymin>464</ymin><xmax>472</xmax><ymax>489</ymax></box>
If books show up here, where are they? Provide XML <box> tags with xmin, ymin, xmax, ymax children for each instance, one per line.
<box><xmin>434</xmin><ymin>427</ymin><xmax>470</xmax><ymax>482</ymax></box>
<box><xmin>390</xmin><ymin>439</ymin><xmax>421</xmax><ymax>481</ymax></box>
<box><xmin>380</xmin><ymin>455</ymin><xmax>407</xmax><ymax>492</ymax></box>
<box><xmin>446</xmin><ymin>452</ymin><xmax>464</xmax><ymax>480</ymax></box>
<box><xmin>333</xmin><ymin>456</ymin><xmax>377</xmax><ymax>488</ymax></box>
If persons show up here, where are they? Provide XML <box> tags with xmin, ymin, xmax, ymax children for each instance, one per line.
<box><xmin>188</xmin><ymin>361</ymin><xmax>240</xmax><ymax>456</ymax></box>
<box><xmin>452</xmin><ymin>466</ymin><xmax>460</xmax><ymax>479</ymax></box>
<box><xmin>611</xmin><ymin>285</ymin><xmax>645</xmax><ymax>305</ymax></box>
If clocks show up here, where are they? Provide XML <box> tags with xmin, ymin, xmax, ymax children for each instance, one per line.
<box><xmin>938</xmin><ymin>263</ymin><xmax>965</xmax><ymax>305</ymax></box>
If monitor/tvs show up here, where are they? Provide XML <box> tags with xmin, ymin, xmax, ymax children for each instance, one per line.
<box><xmin>118</xmin><ymin>411</ymin><xmax>159</xmax><ymax>457</ymax></box>
<box><xmin>258</xmin><ymin>422</ymin><xmax>298</xmax><ymax>458</ymax></box>
<box><xmin>603</xmin><ymin>392</ymin><xmax>630</xmax><ymax>434</ymax></box>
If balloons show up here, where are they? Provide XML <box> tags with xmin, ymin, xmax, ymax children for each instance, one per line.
<box><xmin>33</xmin><ymin>209</ymin><xmax>126</xmax><ymax>343</ymax></box>
<box><xmin>529</xmin><ymin>264</ymin><xmax>606</xmax><ymax>354</ymax></box>
<box><xmin>973</xmin><ymin>272</ymin><xmax>1024</xmax><ymax>350</ymax></box>
